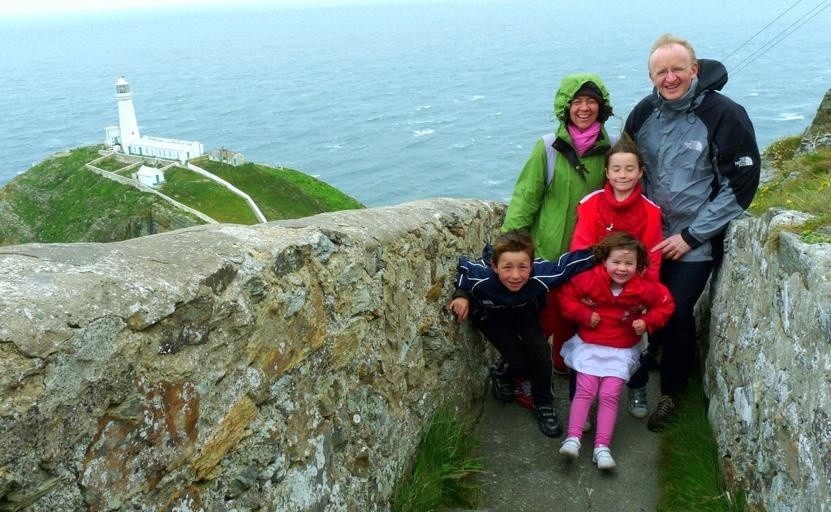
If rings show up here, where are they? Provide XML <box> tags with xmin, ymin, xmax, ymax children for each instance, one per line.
<box><xmin>670</xmin><ymin>251</ymin><xmax>674</xmax><ymax>257</ymax></box>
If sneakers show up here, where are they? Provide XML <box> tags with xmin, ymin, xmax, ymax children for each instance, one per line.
<box><xmin>530</xmin><ymin>403</ymin><xmax>562</xmax><ymax>439</ymax></box>
<box><xmin>490</xmin><ymin>369</ymin><xmax>533</xmax><ymax>410</ymax></box>
<box><xmin>558</xmin><ymin>436</ymin><xmax>582</xmax><ymax>459</ymax></box>
<box><xmin>647</xmin><ymin>392</ymin><xmax>678</xmax><ymax>434</ymax></box>
<box><xmin>592</xmin><ymin>445</ymin><xmax>617</xmax><ymax>471</ymax></box>
<box><xmin>627</xmin><ymin>387</ymin><xmax>650</xmax><ymax>420</ymax></box>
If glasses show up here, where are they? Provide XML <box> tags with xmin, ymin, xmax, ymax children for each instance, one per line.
<box><xmin>655</xmin><ymin>63</ymin><xmax>699</xmax><ymax>78</ymax></box>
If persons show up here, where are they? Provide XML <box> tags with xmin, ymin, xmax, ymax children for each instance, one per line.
<box><xmin>566</xmin><ymin>137</ymin><xmax>666</xmax><ymax>436</ymax></box>
<box><xmin>499</xmin><ymin>70</ymin><xmax>611</xmax><ymax>410</ymax></box>
<box><xmin>448</xmin><ymin>230</ymin><xmax>605</xmax><ymax>439</ymax></box>
<box><xmin>604</xmin><ymin>32</ymin><xmax>762</xmax><ymax>433</ymax></box>
<box><xmin>554</xmin><ymin>233</ymin><xmax>679</xmax><ymax>472</ymax></box>
